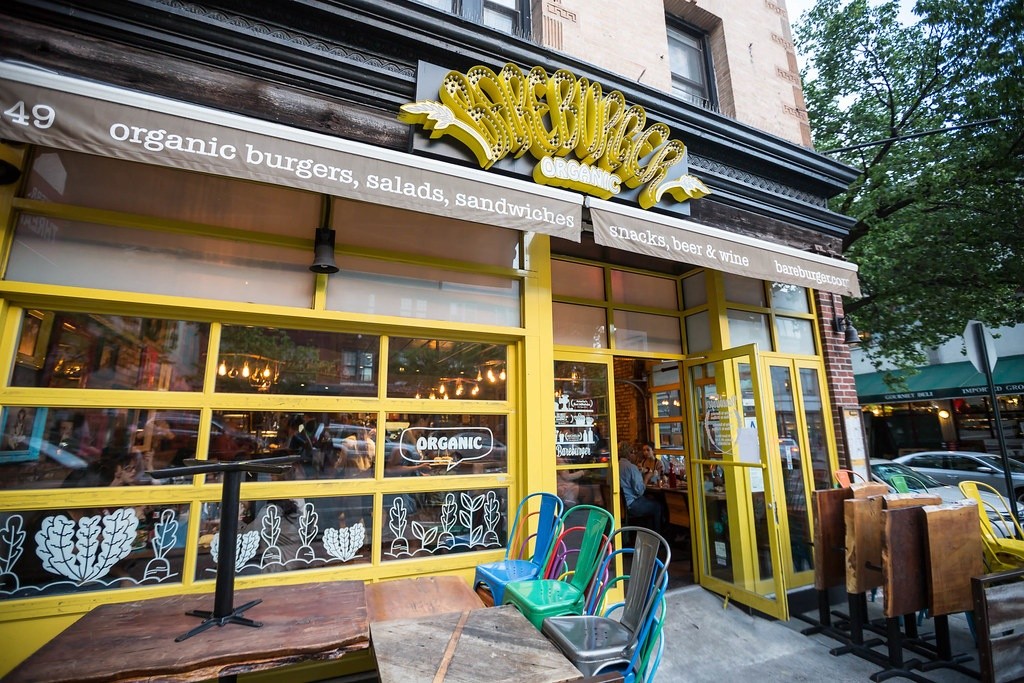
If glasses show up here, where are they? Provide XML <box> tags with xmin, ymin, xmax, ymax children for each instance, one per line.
<box><xmin>123</xmin><ymin>466</ymin><xmax>137</xmax><ymax>472</ymax></box>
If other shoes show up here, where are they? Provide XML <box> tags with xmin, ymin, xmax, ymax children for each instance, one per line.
<box><xmin>675</xmin><ymin>534</ymin><xmax>687</xmax><ymax>542</ymax></box>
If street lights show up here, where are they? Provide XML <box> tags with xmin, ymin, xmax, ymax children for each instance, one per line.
<box><xmin>661</xmin><ymin>399</ymin><xmax>680</xmax><ymax>446</ymax></box>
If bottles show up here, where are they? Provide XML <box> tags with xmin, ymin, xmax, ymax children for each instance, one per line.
<box><xmin>713</xmin><ymin>473</ymin><xmax>722</xmax><ymax>486</ymax></box>
<box><xmin>669</xmin><ymin>463</ymin><xmax>676</xmax><ymax>488</ymax></box>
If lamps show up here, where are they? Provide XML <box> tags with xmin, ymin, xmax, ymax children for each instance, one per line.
<box><xmin>831</xmin><ymin>314</ymin><xmax>864</xmax><ymax>344</ymax></box>
<box><xmin>308</xmin><ymin>192</ymin><xmax>340</xmax><ymax>274</ymax></box>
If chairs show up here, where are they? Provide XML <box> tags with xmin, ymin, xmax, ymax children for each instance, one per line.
<box><xmin>601</xmin><ymin>483</ymin><xmax>649</xmax><ymax>547</ymax></box>
<box><xmin>473</xmin><ymin>490</ymin><xmax>671</xmax><ymax>683</ymax></box>
<box><xmin>832</xmin><ymin>467</ymin><xmax>1024</xmax><ymax>583</ymax></box>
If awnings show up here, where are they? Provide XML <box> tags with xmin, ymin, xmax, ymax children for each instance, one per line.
<box><xmin>854</xmin><ymin>354</ymin><xmax>1024</xmax><ymax>405</ymax></box>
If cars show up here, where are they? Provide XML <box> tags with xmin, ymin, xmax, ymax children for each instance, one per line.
<box><xmin>598</xmin><ymin>451</ymin><xmax>610</xmax><ymax>463</ymax></box>
<box><xmin>869</xmin><ymin>457</ymin><xmax>1024</xmax><ymax>539</ymax></box>
<box><xmin>0</xmin><ymin>427</ymin><xmax>164</xmax><ymax>536</ymax></box>
<box><xmin>203</xmin><ymin>454</ymin><xmax>418</xmax><ymax>529</ymax></box>
<box><xmin>557</xmin><ymin>433</ymin><xmax>610</xmax><ymax>479</ymax></box>
<box><xmin>892</xmin><ymin>451</ymin><xmax>1024</xmax><ymax>505</ymax></box>
<box><xmin>661</xmin><ymin>445</ymin><xmax>684</xmax><ymax>463</ymax></box>
<box><xmin>426</xmin><ymin>431</ymin><xmax>507</xmax><ymax>462</ymax></box>
<box><xmin>779</xmin><ymin>438</ymin><xmax>800</xmax><ymax>468</ymax></box>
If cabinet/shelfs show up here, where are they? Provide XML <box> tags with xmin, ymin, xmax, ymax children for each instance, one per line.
<box><xmin>554</xmin><ymin>408</ymin><xmax>596</xmax><ymax>445</ymax></box>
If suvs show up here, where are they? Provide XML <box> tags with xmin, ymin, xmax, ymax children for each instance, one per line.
<box><xmin>141</xmin><ymin>414</ymin><xmax>267</xmax><ymax>467</ymax></box>
<box><xmin>314</xmin><ymin>424</ymin><xmax>419</xmax><ymax>469</ymax></box>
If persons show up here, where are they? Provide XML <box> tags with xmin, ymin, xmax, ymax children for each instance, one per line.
<box><xmin>5</xmin><ymin>409</ymin><xmax>190</xmax><ymax>593</ymax></box>
<box><xmin>556</xmin><ymin>454</ymin><xmax>592</xmax><ymax>501</ymax></box>
<box><xmin>387</xmin><ymin>414</ymin><xmax>433</xmax><ymax>558</ymax></box>
<box><xmin>222</xmin><ymin>413</ymin><xmax>376</xmax><ymax>575</ymax></box>
<box><xmin>637</xmin><ymin>442</ymin><xmax>665</xmax><ymax>474</ymax></box>
<box><xmin>619</xmin><ymin>441</ymin><xmax>663</xmax><ymax>532</ymax></box>
<box><xmin>562</xmin><ymin>427</ymin><xmax>604</xmax><ymax>451</ymax></box>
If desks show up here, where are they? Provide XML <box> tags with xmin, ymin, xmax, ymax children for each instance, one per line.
<box><xmin>582</xmin><ymin>472</ymin><xmax>727</xmax><ymax>534</ymax></box>
